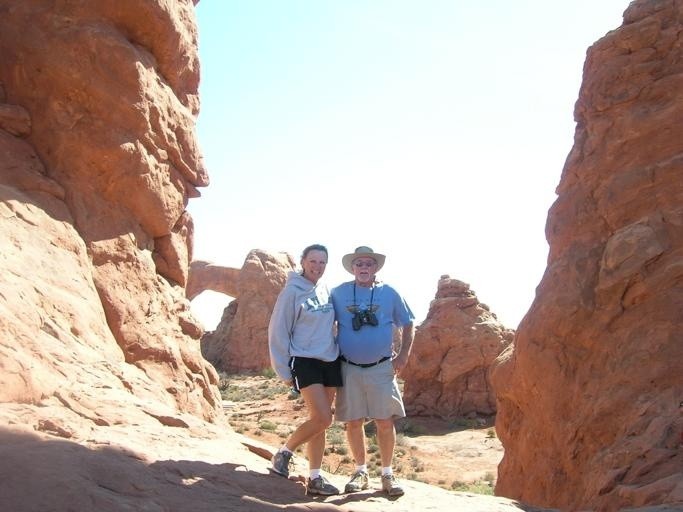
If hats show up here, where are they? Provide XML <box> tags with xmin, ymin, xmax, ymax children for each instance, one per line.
<box><xmin>342</xmin><ymin>246</ymin><xmax>386</xmax><ymax>276</ymax></box>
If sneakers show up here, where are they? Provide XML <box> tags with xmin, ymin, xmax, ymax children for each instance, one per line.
<box><xmin>381</xmin><ymin>474</ymin><xmax>403</xmax><ymax>496</ymax></box>
<box><xmin>307</xmin><ymin>474</ymin><xmax>339</xmax><ymax>495</ymax></box>
<box><xmin>344</xmin><ymin>471</ymin><xmax>370</xmax><ymax>493</ymax></box>
<box><xmin>270</xmin><ymin>449</ymin><xmax>292</xmax><ymax>478</ymax></box>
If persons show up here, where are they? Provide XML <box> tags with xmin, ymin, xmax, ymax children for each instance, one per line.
<box><xmin>269</xmin><ymin>245</ymin><xmax>415</xmax><ymax>495</ymax></box>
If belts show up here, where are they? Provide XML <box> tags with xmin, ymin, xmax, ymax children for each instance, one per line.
<box><xmin>340</xmin><ymin>354</ymin><xmax>390</xmax><ymax>369</ymax></box>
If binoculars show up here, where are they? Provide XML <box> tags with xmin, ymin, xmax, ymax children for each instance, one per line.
<box><xmin>352</xmin><ymin>309</ymin><xmax>378</xmax><ymax>331</ymax></box>
